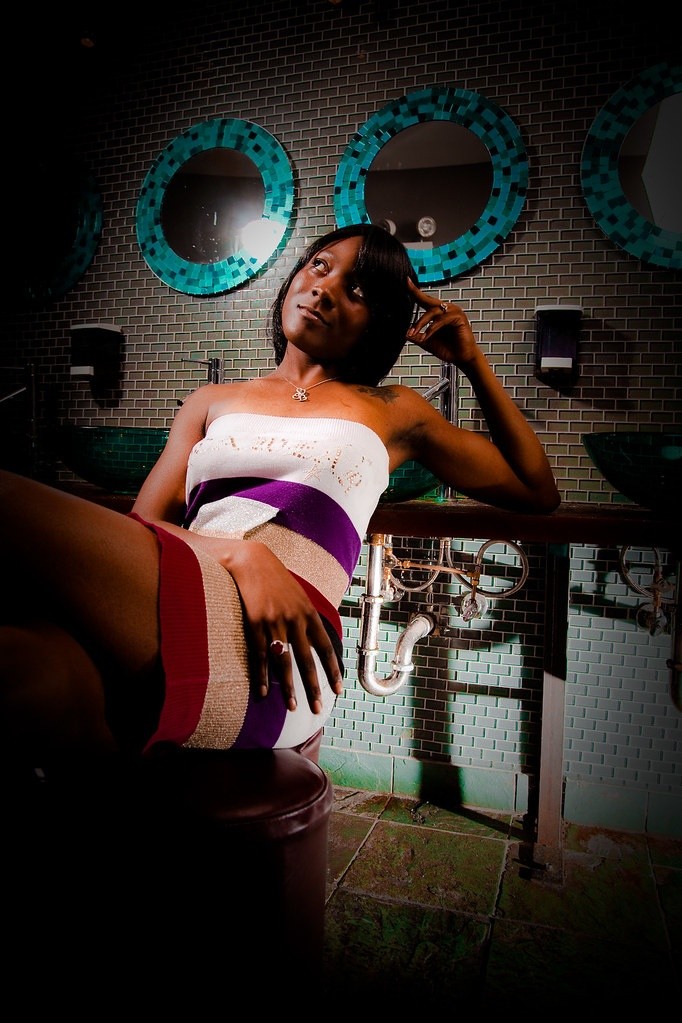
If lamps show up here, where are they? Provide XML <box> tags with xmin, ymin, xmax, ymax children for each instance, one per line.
<box><xmin>382</xmin><ymin>216</ymin><xmax>437</xmax><ymax>250</ymax></box>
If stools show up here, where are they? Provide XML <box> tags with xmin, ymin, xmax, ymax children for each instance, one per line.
<box><xmin>181</xmin><ymin>748</ymin><xmax>327</xmax><ymax>967</ymax></box>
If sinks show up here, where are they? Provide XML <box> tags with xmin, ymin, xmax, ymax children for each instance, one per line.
<box><xmin>380</xmin><ymin>459</ymin><xmax>443</xmax><ymax>505</ymax></box>
<box><xmin>60</xmin><ymin>424</ymin><xmax>172</xmax><ymax>495</ymax></box>
<box><xmin>583</xmin><ymin>430</ymin><xmax>682</xmax><ymax>509</ymax></box>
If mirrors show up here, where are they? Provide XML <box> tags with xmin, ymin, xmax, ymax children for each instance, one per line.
<box><xmin>333</xmin><ymin>87</ymin><xmax>530</xmax><ymax>282</ymax></box>
<box><xmin>580</xmin><ymin>59</ymin><xmax>682</xmax><ymax>269</ymax></box>
<box><xmin>135</xmin><ymin>119</ymin><xmax>293</xmax><ymax>295</ymax></box>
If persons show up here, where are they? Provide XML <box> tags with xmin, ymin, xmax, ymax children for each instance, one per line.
<box><xmin>0</xmin><ymin>225</ymin><xmax>562</xmax><ymax>758</ymax></box>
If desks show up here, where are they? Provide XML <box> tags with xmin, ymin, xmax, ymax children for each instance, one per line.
<box><xmin>55</xmin><ymin>484</ymin><xmax>682</xmax><ymax>549</ymax></box>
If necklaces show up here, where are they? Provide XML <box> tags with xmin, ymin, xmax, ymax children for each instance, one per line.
<box><xmin>276</xmin><ymin>367</ymin><xmax>339</xmax><ymax>402</ymax></box>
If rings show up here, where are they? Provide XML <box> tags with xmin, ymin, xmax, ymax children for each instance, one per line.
<box><xmin>270</xmin><ymin>639</ymin><xmax>289</xmax><ymax>655</ymax></box>
<box><xmin>440</xmin><ymin>302</ymin><xmax>448</xmax><ymax>313</ymax></box>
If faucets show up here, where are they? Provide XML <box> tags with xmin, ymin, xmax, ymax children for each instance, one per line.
<box><xmin>420</xmin><ymin>362</ymin><xmax>459</xmax><ymax>502</ymax></box>
<box><xmin>0</xmin><ymin>361</ymin><xmax>40</xmax><ymax>454</ymax></box>
<box><xmin>176</xmin><ymin>357</ymin><xmax>225</xmax><ymax>407</ymax></box>
